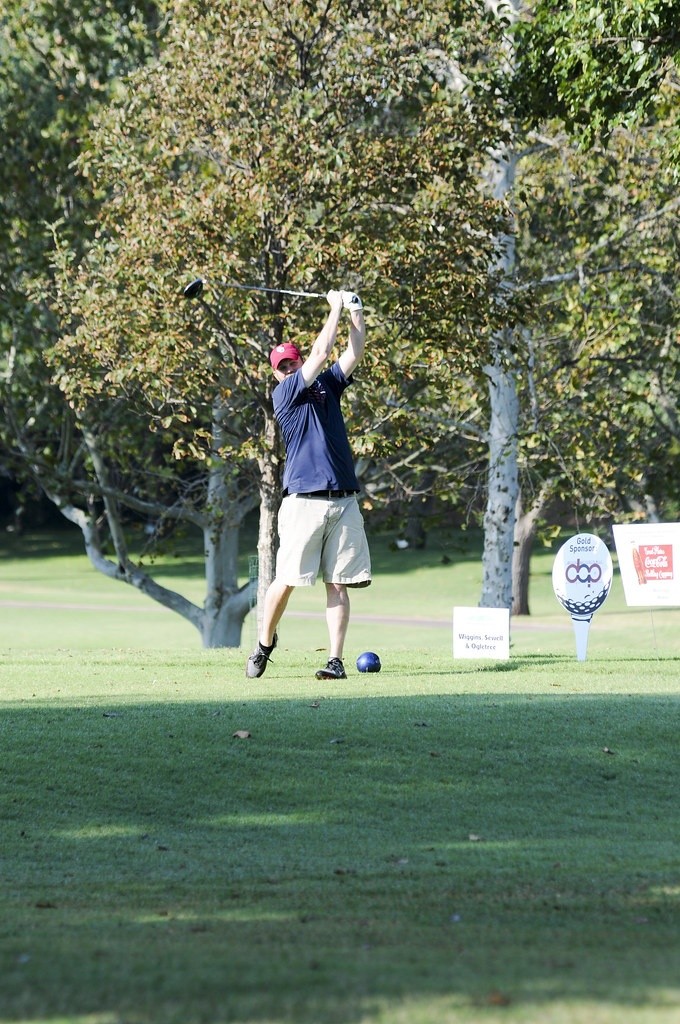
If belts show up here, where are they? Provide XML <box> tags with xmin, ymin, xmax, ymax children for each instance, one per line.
<box><xmin>306</xmin><ymin>490</ymin><xmax>354</xmax><ymax>498</ymax></box>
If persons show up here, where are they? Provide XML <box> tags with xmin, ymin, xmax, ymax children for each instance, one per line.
<box><xmin>246</xmin><ymin>289</ymin><xmax>371</xmax><ymax>679</ymax></box>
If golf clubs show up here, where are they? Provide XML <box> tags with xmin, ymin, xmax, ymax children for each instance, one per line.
<box><xmin>183</xmin><ymin>278</ymin><xmax>358</xmax><ymax>303</ymax></box>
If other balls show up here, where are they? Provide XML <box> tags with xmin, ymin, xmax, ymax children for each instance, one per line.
<box><xmin>356</xmin><ymin>652</ymin><xmax>381</xmax><ymax>673</ymax></box>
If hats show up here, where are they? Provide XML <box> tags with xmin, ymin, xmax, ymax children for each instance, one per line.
<box><xmin>270</xmin><ymin>343</ymin><xmax>302</xmax><ymax>370</ymax></box>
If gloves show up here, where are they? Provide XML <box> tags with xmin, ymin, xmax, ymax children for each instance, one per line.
<box><xmin>340</xmin><ymin>290</ymin><xmax>363</xmax><ymax>312</ymax></box>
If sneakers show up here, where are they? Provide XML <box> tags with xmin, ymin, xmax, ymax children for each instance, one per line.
<box><xmin>315</xmin><ymin>657</ymin><xmax>347</xmax><ymax>680</ymax></box>
<box><xmin>246</xmin><ymin>630</ymin><xmax>278</xmax><ymax>679</ymax></box>
